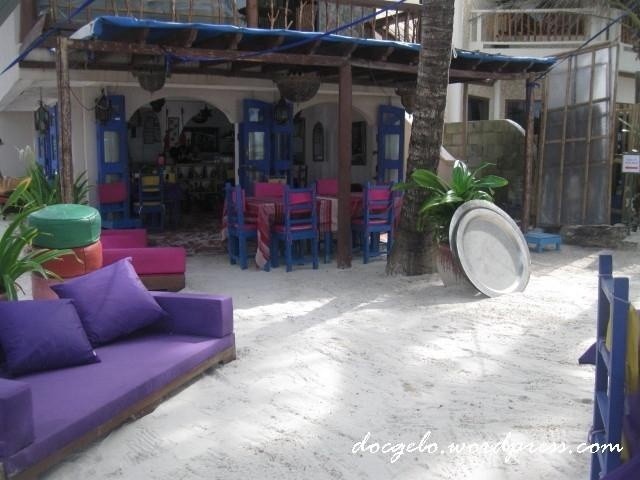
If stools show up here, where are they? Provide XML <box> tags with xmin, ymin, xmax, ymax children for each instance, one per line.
<box><xmin>28</xmin><ymin>204</ymin><xmax>101</xmax><ymax>299</ymax></box>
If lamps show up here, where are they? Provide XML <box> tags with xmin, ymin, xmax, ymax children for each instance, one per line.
<box><xmin>94</xmin><ymin>89</ymin><xmax>112</xmax><ymax>127</ymax></box>
<box><xmin>275</xmin><ymin>96</ymin><xmax>288</xmax><ymax>126</ymax></box>
<box><xmin>394</xmin><ymin>82</ymin><xmax>415</xmax><ymax>116</ymax></box>
<box><xmin>277</xmin><ymin>72</ymin><xmax>321</xmax><ymax>108</ymax></box>
<box><xmin>34</xmin><ymin>87</ymin><xmax>50</xmax><ymax>134</ymax></box>
<box><xmin>132</xmin><ymin>69</ymin><xmax>171</xmax><ymax>94</ymax></box>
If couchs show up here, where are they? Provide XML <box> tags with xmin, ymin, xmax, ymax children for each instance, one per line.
<box><xmin>0</xmin><ymin>290</ymin><xmax>235</xmax><ymax>480</ymax></box>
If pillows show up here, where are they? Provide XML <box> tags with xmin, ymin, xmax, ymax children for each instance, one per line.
<box><xmin>50</xmin><ymin>256</ymin><xmax>172</xmax><ymax>349</ymax></box>
<box><xmin>0</xmin><ymin>301</ymin><xmax>100</xmax><ymax>380</ymax></box>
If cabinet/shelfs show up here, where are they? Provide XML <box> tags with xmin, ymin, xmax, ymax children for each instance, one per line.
<box><xmin>174</xmin><ymin>162</ymin><xmax>225</xmax><ymax>199</ymax></box>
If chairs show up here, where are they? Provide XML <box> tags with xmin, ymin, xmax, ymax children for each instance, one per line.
<box><xmin>226</xmin><ymin>180</ymin><xmax>395</xmax><ymax>272</ymax></box>
<box><xmin>132</xmin><ymin>175</ymin><xmax>165</xmax><ymax>232</ymax></box>
<box><xmin>101</xmin><ymin>229</ymin><xmax>186</xmax><ymax>291</ymax></box>
<box><xmin>591</xmin><ymin>254</ymin><xmax>640</xmax><ymax>480</ymax></box>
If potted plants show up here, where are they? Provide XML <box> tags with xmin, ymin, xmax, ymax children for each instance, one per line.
<box><xmin>390</xmin><ymin>160</ymin><xmax>509</xmax><ymax>296</ymax></box>
<box><xmin>1</xmin><ymin>179</ymin><xmax>85</xmax><ymax>299</ymax></box>
